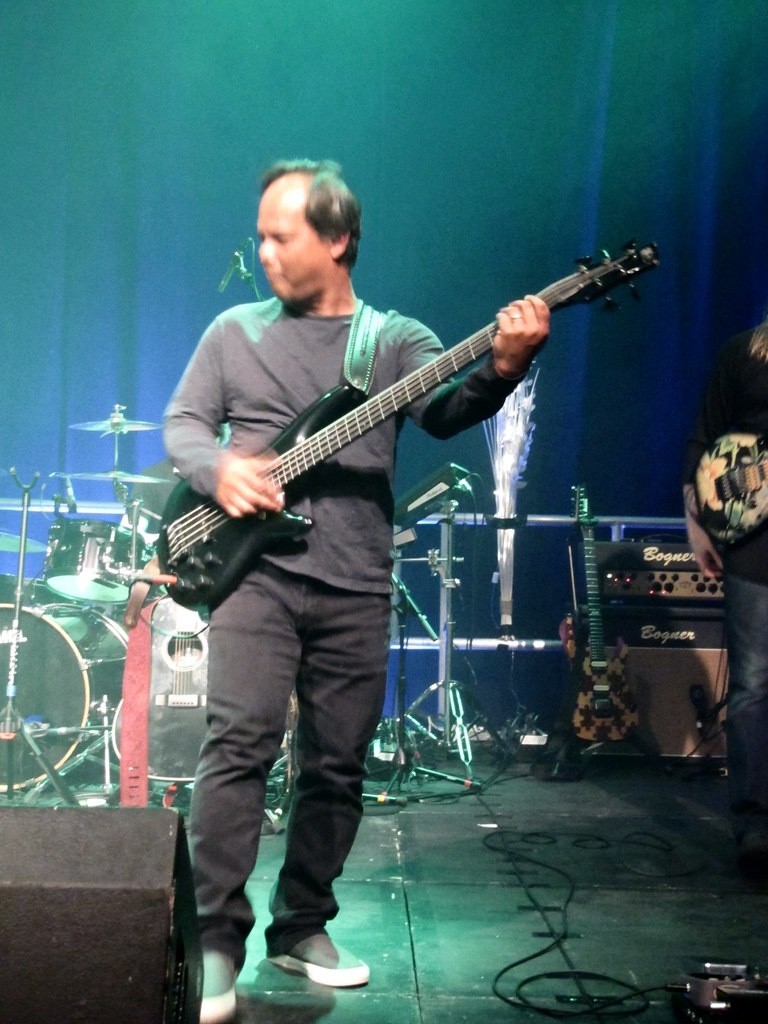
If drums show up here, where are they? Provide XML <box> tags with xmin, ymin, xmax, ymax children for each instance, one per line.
<box><xmin>42</xmin><ymin>516</ymin><xmax>148</xmax><ymax>604</ymax></box>
<box><xmin>0</xmin><ymin>599</ymin><xmax>129</xmax><ymax>790</ymax></box>
<box><xmin>0</xmin><ymin>574</ymin><xmax>45</xmax><ymax>605</ymax></box>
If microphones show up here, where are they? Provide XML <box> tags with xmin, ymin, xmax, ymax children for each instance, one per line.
<box><xmin>65</xmin><ymin>477</ymin><xmax>77</xmax><ymax>514</ymax></box>
<box><xmin>218</xmin><ymin>239</ymin><xmax>249</xmax><ymax>292</ymax></box>
<box><xmin>690</xmin><ymin>683</ymin><xmax>706</xmax><ymax>735</ymax></box>
<box><xmin>24</xmin><ymin>721</ymin><xmax>45</xmax><ymax>740</ymax></box>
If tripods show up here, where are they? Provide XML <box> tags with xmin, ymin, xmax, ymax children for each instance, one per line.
<box><xmin>360</xmin><ymin>495</ymin><xmax>508</xmax><ymax>804</ymax></box>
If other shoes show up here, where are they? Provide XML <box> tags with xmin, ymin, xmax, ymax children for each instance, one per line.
<box><xmin>200</xmin><ymin>949</ymin><xmax>236</xmax><ymax>1024</ymax></box>
<box><xmin>267</xmin><ymin>928</ymin><xmax>369</xmax><ymax>987</ymax></box>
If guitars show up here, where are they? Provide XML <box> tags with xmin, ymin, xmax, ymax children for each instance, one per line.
<box><xmin>689</xmin><ymin>429</ymin><xmax>768</xmax><ymax>542</ymax></box>
<box><xmin>152</xmin><ymin>240</ymin><xmax>661</xmax><ymax>617</ymax></box>
<box><xmin>559</xmin><ymin>475</ymin><xmax>639</xmax><ymax>746</ymax></box>
<box><xmin>110</xmin><ymin>593</ymin><xmax>210</xmax><ymax>784</ymax></box>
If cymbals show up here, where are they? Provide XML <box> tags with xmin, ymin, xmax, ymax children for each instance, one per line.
<box><xmin>49</xmin><ymin>467</ymin><xmax>171</xmax><ymax>485</ymax></box>
<box><xmin>0</xmin><ymin>531</ymin><xmax>47</xmax><ymax>552</ymax></box>
<box><xmin>67</xmin><ymin>418</ymin><xmax>164</xmax><ymax>432</ymax></box>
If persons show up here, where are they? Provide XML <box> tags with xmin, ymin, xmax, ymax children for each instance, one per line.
<box><xmin>128</xmin><ymin>419</ymin><xmax>227</xmax><ymax>542</ymax></box>
<box><xmin>681</xmin><ymin>316</ymin><xmax>768</xmax><ymax>850</ymax></box>
<box><xmin>161</xmin><ymin>157</ymin><xmax>550</xmax><ymax>1024</ymax></box>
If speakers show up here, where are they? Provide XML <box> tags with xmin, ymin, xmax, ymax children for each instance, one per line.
<box><xmin>563</xmin><ymin>605</ymin><xmax>730</xmax><ymax>765</ymax></box>
<box><xmin>0</xmin><ymin>805</ymin><xmax>205</xmax><ymax>1024</ymax></box>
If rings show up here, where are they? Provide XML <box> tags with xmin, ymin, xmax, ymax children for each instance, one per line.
<box><xmin>511</xmin><ymin>312</ymin><xmax>521</xmax><ymax>319</ymax></box>
